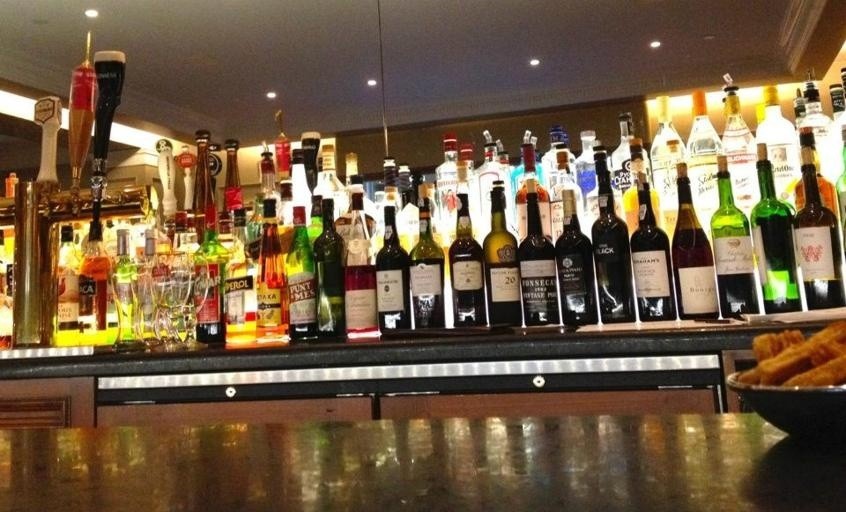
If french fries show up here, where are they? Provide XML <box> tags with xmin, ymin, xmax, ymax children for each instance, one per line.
<box><xmin>736</xmin><ymin>320</ymin><xmax>845</xmax><ymax>387</ymax></box>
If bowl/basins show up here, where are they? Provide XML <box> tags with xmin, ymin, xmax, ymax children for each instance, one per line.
<box><xmin>726</xmin><ymin>368</ymin><xmax>846</xmax><ymax>439</ymax></box>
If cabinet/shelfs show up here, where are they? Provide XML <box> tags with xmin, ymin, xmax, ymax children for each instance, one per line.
<box><xmin>0</xmin><ymin>305</ymin><xmax>846</xmax><ymax>430</ymax></box>
